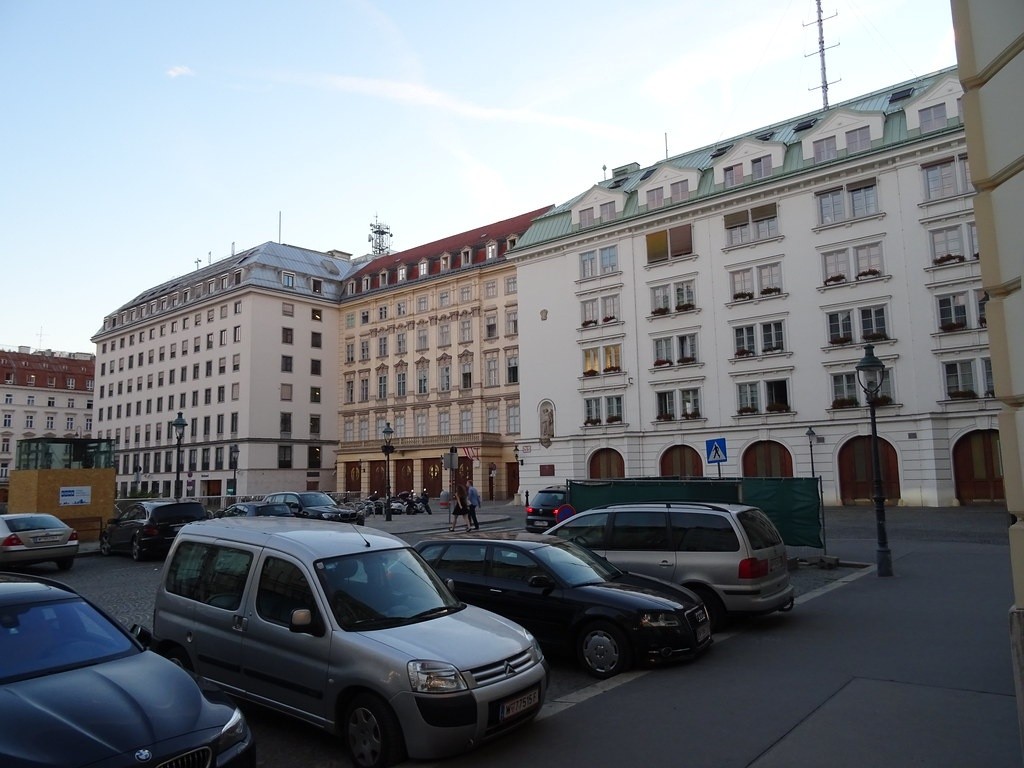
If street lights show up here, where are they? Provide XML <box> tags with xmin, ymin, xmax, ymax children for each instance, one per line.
<box><xmin>230</xmin><ymin>444</ymin><xmax>240</xmax><ymax>498</ymax></box>
<box><xmin>381</xmin><ymin>421</ymin><xmax>394</xmax><ymax>522</ymax></box>
<box><xmin>805</xmin><ymin>425</ymin><xmax>816</xmax><ymax>478</ymax></box>
<box><xmin>854</xmin><ymin>343</ymin><xmax>894</xmax><ymax>578</ymax></box>
<box><xmin>171</xmin><ymin>412</ymin><xmax>189</xmax><ymax>505</ymax></box>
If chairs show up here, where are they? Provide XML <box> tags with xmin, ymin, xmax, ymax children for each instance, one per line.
<box><xmin>335</xmin><ymin>560</ymin><xmax>364</xmax><ymax>604</ymax></box>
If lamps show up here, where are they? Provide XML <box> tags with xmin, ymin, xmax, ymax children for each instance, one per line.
<box><xmin>358</xmin><ymin>459</ymin><xmax>365</xmax><ymax>472</ymax></box>
<box><xmin>514</xmin><ymin>445</ymin><xmax>524</xmax><ymax>466</ymax></box>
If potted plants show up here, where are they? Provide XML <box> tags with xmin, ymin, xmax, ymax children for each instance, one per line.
<box><xmin>584</xmin><ymin>419</ymin><xmax>601</xmax><ymax>426</ymax></box>
<box><xmin>583</xmin><ymin>370</ymin><xmax>598</xmax><ymax>377</ymax></box>
<box><xmin>832</xmin><ymin>398</ymin><xmax>859</xmax><ymax>409</ymax></box>
<box><xmin>932</xmin><ymin>255</ymin><xmax>965</xmax><ymax>265</ymax></box>
<box><xmin>824</xmin><ymin>274</ymin><xmax>847</xmax><ymax>286</ymax></box>
<box><xmin>876</xmin><ymin>396</ymin><xmax>893</xmax><ymax>407</ymax></box>
<box><xmin>828</xmin><ymin>337</ymin><xmax>851</xmax><ymax>346</ymax></box>
<box><xmin>607</xmin><ymin>416</ymin><xmax>622</xmax><ymax>425</ymax></box>
<box><xmin>735</xmin><ymin>347</ymin><xmax>754</xmax><ymax>359</ymax></box>
<box><xmin>737</xmin><ymin>406</ymin><xmax>759</xmax><ymax>416</ymax></box>
<box><xmin>939</xmin><ymin>322</ymin><xmax>967</xmax><ymax>331</ymax></box>
<box><xmin>651</xmin><ymin>308</ymin><xmax>669</xmax><ymax>316</ymax></box>
<box><xmin>682</xmin><ymin>412</ymin><xmax>701</xmax><ymax>419</ymax></box>
<box><xmin>766</xmin><ymin>403</ymin><xmax>790</xmax><ymax>413</ymax></box>
<box><xmin>856</xmin><ymin>268</ymin><xmax>880</xmax><ymax>281</ymax></box>
<box><xmin>762</xmin><ymin>345</ymin><xmax>782</xmax><ymax>355</ymax></box>
<box><xmin>676</xmin><ymin>303</ymin><xmax>695</xmax><ymax>312</ymax></box>
<box><xmin>945</xmin><ymin>389</ymin><xmax>977</xmax><ymax>400</ymax></box>
<box><xmin>760</xmin><ymin>287</ymin><xmax>781</xmax><ymax>297</ymax></box>
<box><xmin>654</xmin><ymin>359</ymin><xmax>673</xmax><ymax>368</ymax></box>
<box><xmin>862</xmin><ymin>333</ymin><xmax>888</xmax><ymax>343</ymax></box>
<box><xmin>603</xmin><ymin>316</ymin><xmax>617</xmax><ymax>324</ymax></box>
<box><xmin>733</xmin><ymin>291</ymin><xmax>754</xmax><ymax>302</ymax></box>
<box><xmin>677</xmin><ymin>357</ymin><xmax>695</xmax><ymax>365</ymax></box>
<box><xmin>985</xmin><ymin>389</ymin><xmax>995</xmax><ymax>397</ymax></box>
<box><xmin>978</xmin><ymin>316</ymin><xmax>987</xmax><ymax>327</ymax></box>
<box><xmin>656</xmin><ymin>413</ymin><xmax>672</xmax><ymax>421</ymax></box>
<box><xmin>603</xmin><ymin>366</ymin><xmax>621</xmax><ymax>374</ymax></box>
<box><xmin>582</xmin><ymin>320</ymin><xmax>598</xmax><ymax>328</ymax></box>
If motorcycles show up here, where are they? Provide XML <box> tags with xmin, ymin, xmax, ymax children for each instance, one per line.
<box><xmin>364</xmin><ymin>489</ymin><xmax>433</xmax><ymax>514</ymax></box>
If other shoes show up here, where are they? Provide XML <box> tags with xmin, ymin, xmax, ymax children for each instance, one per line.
<box><xmin>473</xmin><ymin>527</ymin><xmax>479</xmax><ymax>530</ymax></box>
<box><xmin>449</xmin><ymin>527</ymin><xmax>454</xmax><ymax>532</ymax></box>
<box><xmin>466</xmin><ymin>528</ymin><xmax>471</xmax><ymax>533</ymax></box>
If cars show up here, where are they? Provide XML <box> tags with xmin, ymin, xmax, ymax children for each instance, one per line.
<box><xmin>0</xmin><ymin>572</ymin><xmax>259</xmax><ymax>768</ymax></box>
<box><xmin>216</xmin><ymin>501</ymin><xmax>296</xmax><ymax>520</ymax></box>
<box><xmin>0</xmin><ymin>513</ymin><xmax>79</xmax><ymax>575</ymax></box>
<box><xmin>384</xmin><ymin>534</ymin><xmax>714</xmax><ymax>681</ymax></box>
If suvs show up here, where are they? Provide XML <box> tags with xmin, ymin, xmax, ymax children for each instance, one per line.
<box><xmin>515</xmin><ymin>502</ymin><xmax>797</xmax><ymax>636</ymax></box>
<box><xmin>524</xmin><ymin>485</ymin><xmax>570</xmax><ymax>534</ymax></box>
<box><xmin>264</xmin><ymin>490</ymin><xmax>358</xmax><ymax>524</ymax></box>
<box><xmin>100</xmin><ymin>500</ymin><xmax>207</xmax><ymax>563</ymax></box>
<box><xmin>152</xmin><ymin>514</ymin><xmax>552</xmax><ymax>768</ymax></box>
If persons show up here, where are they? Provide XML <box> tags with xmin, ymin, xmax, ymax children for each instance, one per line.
<box><xmin>449</xmin><ymin>484</ymin><xmax>473</xmax><ymax>533</ymax></box>
<box><xmin>465</xmin><ymin>480</ymin><xmax>481</xmax><ymax>530</ymax></box>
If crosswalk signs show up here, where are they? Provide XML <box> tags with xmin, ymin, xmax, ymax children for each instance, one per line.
<box><xmin>705</xmin><ymin>438</ymin><xmax>727</xmax><ymax>464</ymax></box>
